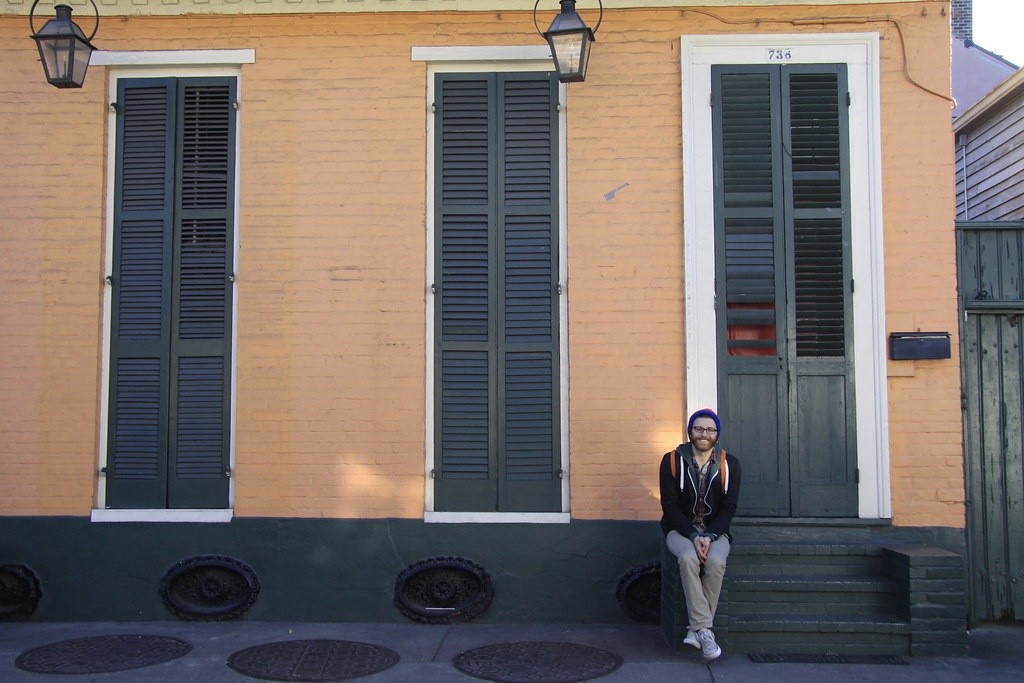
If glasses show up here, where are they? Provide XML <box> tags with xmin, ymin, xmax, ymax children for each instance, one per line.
<box><xmin>692</xmin><ymin>426</ymin><xmax>717</xmax><ymax>435</ymax></box>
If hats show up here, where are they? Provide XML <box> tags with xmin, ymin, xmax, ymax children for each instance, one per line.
<box><xmin>688</xmin><ymin>409</ymin><xmax>721</xmax><ymax>442</ymax></box>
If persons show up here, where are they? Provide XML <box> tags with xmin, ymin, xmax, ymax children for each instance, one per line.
<box><xmin>659</xmin><ymin>408</ymin><xmax>743</xmax><ymax>660</ymax></box>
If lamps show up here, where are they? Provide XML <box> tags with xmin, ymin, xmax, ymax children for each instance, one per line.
<box><xmin>28</xmin><ymin>0</ymin><xmax>99</xmax><ymax>87</ymax></box>
<box><xmin>533</xmin><ymin>0</ymin><xmax>603</xmax><ymax>84</ymax></box>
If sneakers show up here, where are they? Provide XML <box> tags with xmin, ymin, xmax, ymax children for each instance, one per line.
<box><xmin>695</xmin><ymin>630</ymin><xmax>721</xmax><ymax>660</ymax></box>
<box><xmin>684</xmin><ymin>626</ymin><xmax>701</xmax><ymax>650</ymax></box>
